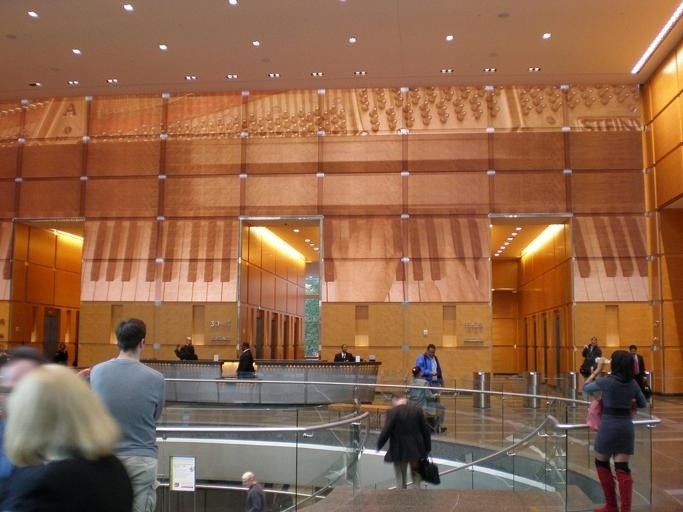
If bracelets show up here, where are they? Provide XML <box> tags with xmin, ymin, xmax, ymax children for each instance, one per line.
<box><xmin>592</xmin><ymin>371</ymin><xmax>598</xmax><ymax>377</ymax></box>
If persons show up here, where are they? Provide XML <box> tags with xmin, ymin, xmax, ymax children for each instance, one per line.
<box><xmin>581</xmin><ymin>348</ymin><xmax>651</xmax><ymax>512</ymax></box>
<box><xmin>374</xmin><ymin>390</ymin><xmax>432</xmax><ymax>490</ymax></box>
<box><xmin>232</xmin><ymin>341</ymin><xmax>257</xmax><ymax>379</ymax></box>
<box><xmin>415</xmin><ymin>342</ymin><xmax>445</xmax><ymax>402</ymax></box>
<box><xmin>0</xmin><ymin>346</ymin><xmax>46</xmax><ymax>482</ymax></box>
<box><xmin>331</xmin><ymin>343</ymin><xmax>356</xmax><ymax>362</ymax></box>
<box><xmin>87</xmin><ymin>316</ymin><xmax>167</xmax><ymax>511</ymax></box>
<box><xmin>172</xmin><ymin>335</ymin><xmax>198</xmax><ymax>361</ymax></box>
<box><xmin>578</xmin><ymin>335</ymin><xmax>603</xmax><ymax>399</ymax></box>
<box><xmin>54</xmin><ymin>342</ymin><xmax>69</xmax><ymax>366</ymax></box>
<box><xmin>406</xmin><ymin>364</ymin><xmax>448</xmax><ymax>432</ymax></box>
<box><xmin>239</xmin><ymin>471</ymin><xmax>268</xmax><ymax>511</ymax></box>
<box><xmin>628</xmin><ymin>344</ymin><xmax>647</xmax><ymax>409</ymax></box>
<box><xmin>272</xmin><ymin>484</ymin><xmax>293</xmax><ymax>508</ymax></box>
<box><xmin>0</xmin><ymin>361</ymin><xmax>136</xmax><ymax>511</ymax></box>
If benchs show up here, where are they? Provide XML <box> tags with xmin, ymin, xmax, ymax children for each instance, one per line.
<box><xmin>328</xmin><ymin>403</ymin><xmax>441</xmax><ymax>435</ymax></box>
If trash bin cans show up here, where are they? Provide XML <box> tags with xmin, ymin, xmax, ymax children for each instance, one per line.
<box><xmin>567</xmin><ymin>371</ymin><xmax>579</xmax><ymax>408</ymax></box>
<box><xmin>473</xmin><ymin>371</ymin><xmax>490</xmax><ymax>409</ymax></box>
<box><xmin>527</xmin><ymin>371</ymin><xmax>540</xmax><ymax>408</ymax></box>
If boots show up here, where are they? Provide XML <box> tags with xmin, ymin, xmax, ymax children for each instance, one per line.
<box><xmin>615</xmin><ymin>469</ymin><xmax>634</xmax><ymax>512</ymax></box>
<box><xmin>592</xmin><ymin>467</ymin><xmax>618</xmax><ymax>512</ymax></box>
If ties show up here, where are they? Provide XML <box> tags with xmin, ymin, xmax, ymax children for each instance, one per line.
<box><xmin>634</xmin><ymin>359</ymin><xmax>639</xmax><ymax>375</ymax></box>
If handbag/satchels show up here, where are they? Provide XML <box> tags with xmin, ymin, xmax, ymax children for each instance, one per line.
<box><xmin>421</xmin><ymin>463</ymin><xmax>440</xmax><ymax>485</ymax></box>
<box><xmin>588</xmin><ymin>400</ymin><xmax>637</xmax><ymax>433</ymax></box>
<box><xmin>644</xmin><ymin>385</ymin><xmax>652</xmax><ymax>397</ymax></box>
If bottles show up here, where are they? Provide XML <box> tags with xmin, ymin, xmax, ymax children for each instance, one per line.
<box><xmin>595</xmin><ymin>357</ymin><xmax>611</xmax><ymax>364</ymax></box>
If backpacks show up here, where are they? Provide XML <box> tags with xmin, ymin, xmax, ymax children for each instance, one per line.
<box><xmin>580</xmin><ymin>345</ymin><xmax>596</xmax><ymax>376</ymax></box>
